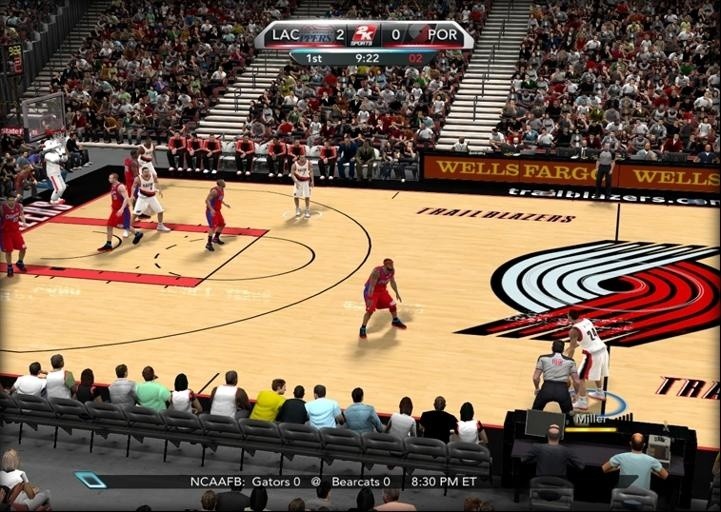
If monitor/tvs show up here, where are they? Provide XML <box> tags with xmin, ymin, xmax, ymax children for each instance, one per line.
<box><xmin>524</xmin><ymin>409</ymin><xmax>566</xmax><ymax>441</ymax></box>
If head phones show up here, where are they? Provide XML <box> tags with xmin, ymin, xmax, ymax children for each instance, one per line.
<box><xmin>545</xmin><ymin>427</ymin><xmax>563</xmax><ymax>442</ymax></box>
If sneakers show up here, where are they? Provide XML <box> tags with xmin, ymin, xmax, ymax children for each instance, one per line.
<box><xmin>360</xmin><ymin>328</ymin><xmax>366</xmax><ymax>338</ymax></box>
<box><xmin>123</xmin><ymin>229</ymin><xmax>143</xmax><ymax>244</ymax></box>
<box><xmin>304</xmin><ymin>210</ymin><xmax>310</xmax><ymax>218</ymax></box>
<box><xmin>392</xmin><ymin>320</ymin><xmax>406</xmax><ymax>329</ymax></box>
<box><xmin>16</xmin><ymin>263</ymin><xmax>26</xmax><ymax>272</ymax></box>
<box><xmin>588</xmin><ymin>392</ymin><xmax>605</xmax><ymax>400</ymax></box>
<box><xmin>212</xmin><ymin>237</ymin><xmax>224</xmax><ymax>245</ymax></box>
<box><xmin>296</xmin><ymin>208</ymin><xmax>301</xmax><ymax>216</ymax></box>
<box><xmin>7</xmin><ymin>268</ymin><xmax>13</xmax><ymax>277</ymax></box>
<box><xmin>572</xmin><ymin>401</ymin><xmax>588</xmax><ymax>410</ymax></box>
<box><xmin>157</xmin><ymin>225</ymin><xmax>171</xmax><ymax>232</ymax></box>
<box><xmin>98</xmin><ymin>244</ymin><xmax>112</xmax><ymax>251</ymax></box>
<box><xmin>206</xmin><ymin>243</ymin><xmax>214</xmax><ymax>251</ymax></box>
